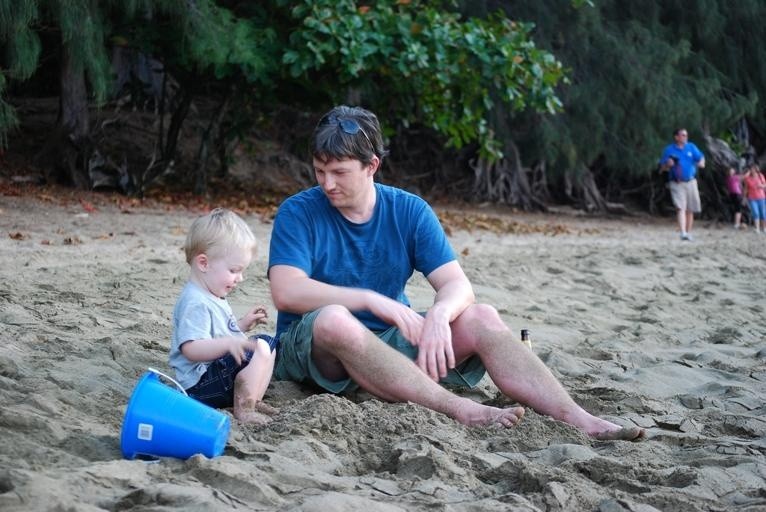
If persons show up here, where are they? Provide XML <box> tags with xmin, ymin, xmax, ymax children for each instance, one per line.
<box><xmin>167</xmin><ymin>207</ymin><xmax>282</xmax><ymax>428</ymax></box>
<box><xmin>659</xmin><ymin>126</ymin><xmax>708</xmax><ymax>241</ymax></box>
<box><xmin>726</xmin><ymin>165</ymin><xmax>752</xmax><ymax>229</ymax></box>
<box><xmin>740</xmin><ymin>161</ymin><xmax>766</xmax><ymax>233</ymax></box>
<box><xmin>264</xmin><ymin>102</ymin><xmax>648</xmax><ymax>441</ymax></box>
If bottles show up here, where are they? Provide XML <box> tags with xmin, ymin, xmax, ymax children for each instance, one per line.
<box><xmin>520</xmin><ymin>329</ymin><xmax>532</xmax><ymax>352</ymax></box>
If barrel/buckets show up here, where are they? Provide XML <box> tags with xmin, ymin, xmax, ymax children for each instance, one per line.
<box><xmin>120</xmin><ymin>366</ymin><xmax>230</xmax><ymax>462</ymax></box>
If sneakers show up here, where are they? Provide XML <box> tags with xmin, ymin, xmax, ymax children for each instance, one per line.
<box><xmin>681</xmin><ymin>231</ymin><xmax>695</xmax><ymax>240</ymax></box>
<box><xmin>753</xmin><ymin>228</ymin><xmax>766</xmax><ymax>234</ymax></box>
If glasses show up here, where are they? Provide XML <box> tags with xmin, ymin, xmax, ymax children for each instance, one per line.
<box><xmin>314</xmin><ymin>110</ymin><xmax>376</xmax><ymax>152</ymax></box>
